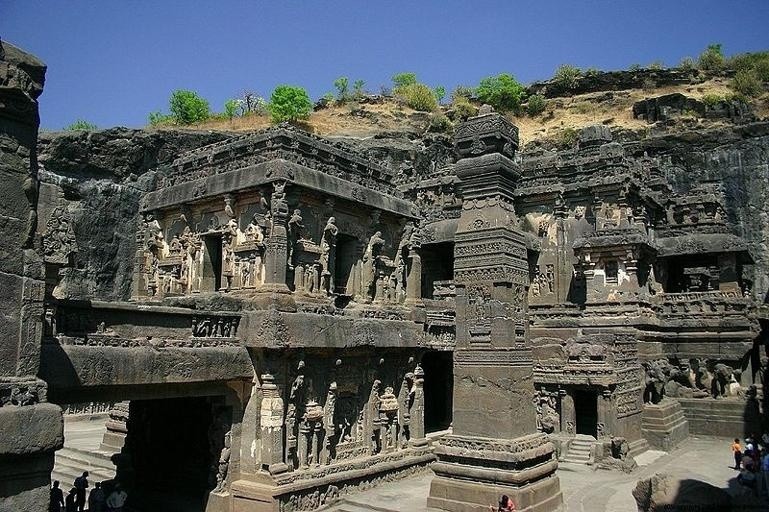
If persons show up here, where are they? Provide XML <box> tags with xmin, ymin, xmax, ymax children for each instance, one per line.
<box><xmin>48</xmin><ymin>470</ymin><xmax>128</xmax><ymax>511</ymax></box>
<box><xmin>498</xmin><ymin>495</ymin><xmax>516</xmax><ymax>511</ymax></box>
<box><xmin>732</xmin><ymin>430</ymin><xmax>769</xmax><ymax>492</ymax></box>
